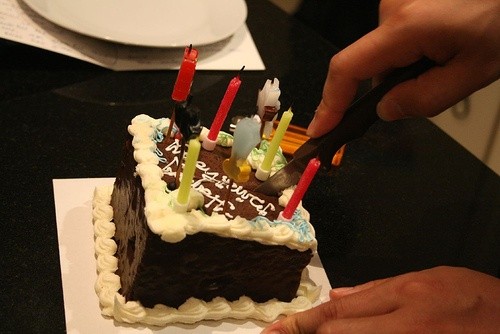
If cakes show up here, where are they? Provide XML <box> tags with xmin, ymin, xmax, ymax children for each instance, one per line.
<box><xmin>90</xmin><ymin>113</ymin><xmax>325</xmax><ymax>327</ymax></box>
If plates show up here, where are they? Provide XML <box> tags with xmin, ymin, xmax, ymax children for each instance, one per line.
<box><xmin>23</xmin><ymin>0</ymin><xmax>248</xmax><ymax>48</ymax></box>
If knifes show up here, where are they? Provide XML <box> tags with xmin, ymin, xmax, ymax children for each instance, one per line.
<box><xmin>254</xmin><ymin>56</ymin><xmax>444</xmax><ymax>197</ymax></box>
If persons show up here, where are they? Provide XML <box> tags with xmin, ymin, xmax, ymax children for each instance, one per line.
<box><xmin>258</xmin><ymin>0</ymin><xmax>499</xmax><ymax>334</ymax></box>
